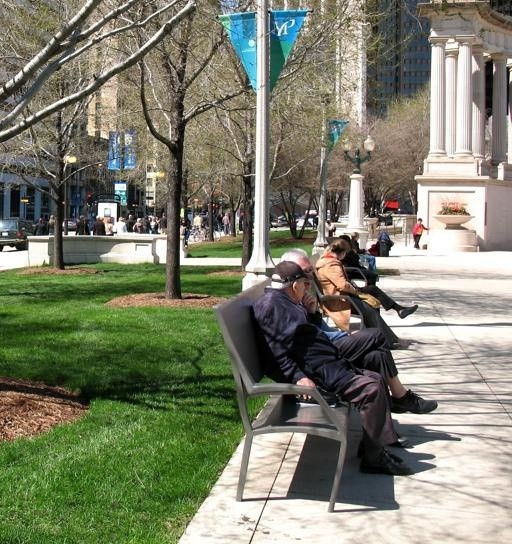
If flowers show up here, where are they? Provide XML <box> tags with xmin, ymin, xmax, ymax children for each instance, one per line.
<box><xmin>437</xmin><ymin>199</ymin><xmax>470</xmax><ymax>214</ymax></box>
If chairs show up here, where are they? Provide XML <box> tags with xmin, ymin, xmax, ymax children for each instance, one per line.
<box><xmin>212</xmin><ymin>266</ymin><xmax>367</xmax><ymax>513</ymax></box>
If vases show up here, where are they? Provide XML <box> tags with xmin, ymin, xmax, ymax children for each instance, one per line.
<box><xmin>432</xmin><ymin>214</ymin><xmax>475</xmax><ymax>230</ymax></box>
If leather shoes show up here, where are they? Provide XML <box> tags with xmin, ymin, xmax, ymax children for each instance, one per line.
<box><xmin>356</xmin><ymin>438</ymin><xmax>415</xmax><ymax>475</ymax></box>
<box><xmin>398</xmin><ymin>305</ymin><xmax>418</xmax><ymax>319</ymax></box>
<box><xmin>383</xmin><ymin>432</ymin><xmax>409</xmax><ymax>448</ymax></box>
<box><xmin>388</xmin><ymin>389</ymin><xmax>439</xmax><ymax>414</ymax></box>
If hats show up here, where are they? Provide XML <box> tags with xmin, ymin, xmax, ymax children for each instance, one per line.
<box><xmin>271</xmin><ymin>259</ymin><xmax>314</xmax><ymax>283</ymax></box>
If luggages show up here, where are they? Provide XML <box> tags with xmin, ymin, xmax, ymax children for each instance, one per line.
<box><xmin>378</xmin><ymin>241</ymin><xmax>389</xmax><ymax>257</ymax></box>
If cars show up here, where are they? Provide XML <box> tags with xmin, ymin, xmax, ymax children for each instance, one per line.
<box><xmin>270</xmin><ymin>206</ymin><xmax>337</xmax><ymax>227</ymax></box>
<box><xmin>62</xmin><ymin>218</ymin><xmax>81</xmax><ymax>231</ymax></box>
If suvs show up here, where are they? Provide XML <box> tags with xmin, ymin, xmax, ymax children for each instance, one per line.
<box><xmin>0</xmin><ymin>217</ymin><xmax>35</xmax><ymax>250</ymax></box>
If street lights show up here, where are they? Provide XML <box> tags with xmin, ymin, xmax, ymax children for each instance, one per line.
<box><xmin>341</xmin><ymin>134</ymin><xmax>375</xmax><ymax>250</ymax></box>
<box><xmin>151</xmin><ymin>170</ymin><xmax>165</xmax><ymax>215</ymax></box>
<box><xmin>61</xmin><ymin>153</ymin><xmax>77</xmax><ymax>235</ymax></box>
<box><xmin>21</xmin><ymin>193</ymin><xmax>29</xmax><ymax>218</ymax></box>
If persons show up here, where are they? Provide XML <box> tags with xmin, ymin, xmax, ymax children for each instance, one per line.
<box><xmin>316</xmin><ymin>219</ymin><xmax>431</xmax><ymax>350</ymax></box>
<box><xmin>252</xmin><ymin>260</ymin><xmax>412</xmax><ymax>475</ymax></box>
<box><xmin>281</xmin><ymin>249</ymin><xmax>437</xmax><ymax>438</ymax></box>
<box><xmin>33</xmin><ymin>213</ymin><xmax>230</xmax><ymax>240</ymax></box>
